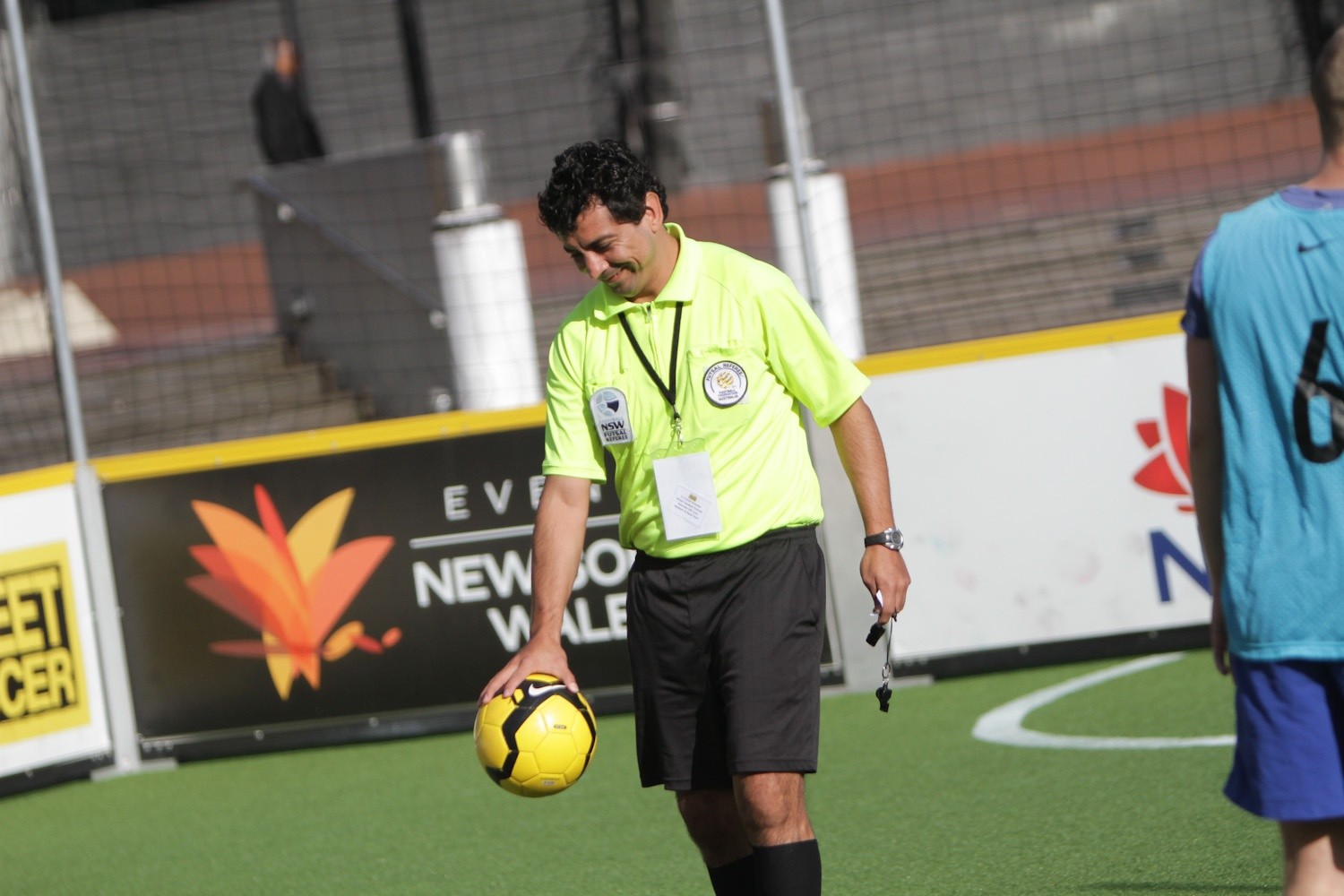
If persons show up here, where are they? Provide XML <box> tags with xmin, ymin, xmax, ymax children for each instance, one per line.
<box><xmin>1180</xmin><ymin>20</ymin><xmax>1344</xmax><ymax>893</ymax></box>
<box><xmin>479</xmin><ymin>139</ymin><xmax>911</xmax><ymax>895</ymax></box>
<box><xmin>250</xmin><ymin>36</ymin><xmax>325</xmax><ymax>165</ymax></box>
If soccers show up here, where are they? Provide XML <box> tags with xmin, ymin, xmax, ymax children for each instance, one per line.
<box><xmin>472</xmin><ymin>669</ymin><xmax>598</xmax><ymax>798</ymax></box>
<box><xmin>593</xmin><ymin>391</ymin><xmax>621</xmax><ymax>418</ymax></box>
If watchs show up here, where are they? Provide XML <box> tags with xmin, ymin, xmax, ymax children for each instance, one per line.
<box><xmin>864</xmin><ymin>527</ymin><xmax>905</xmax><ymax>551</ymax></box>
